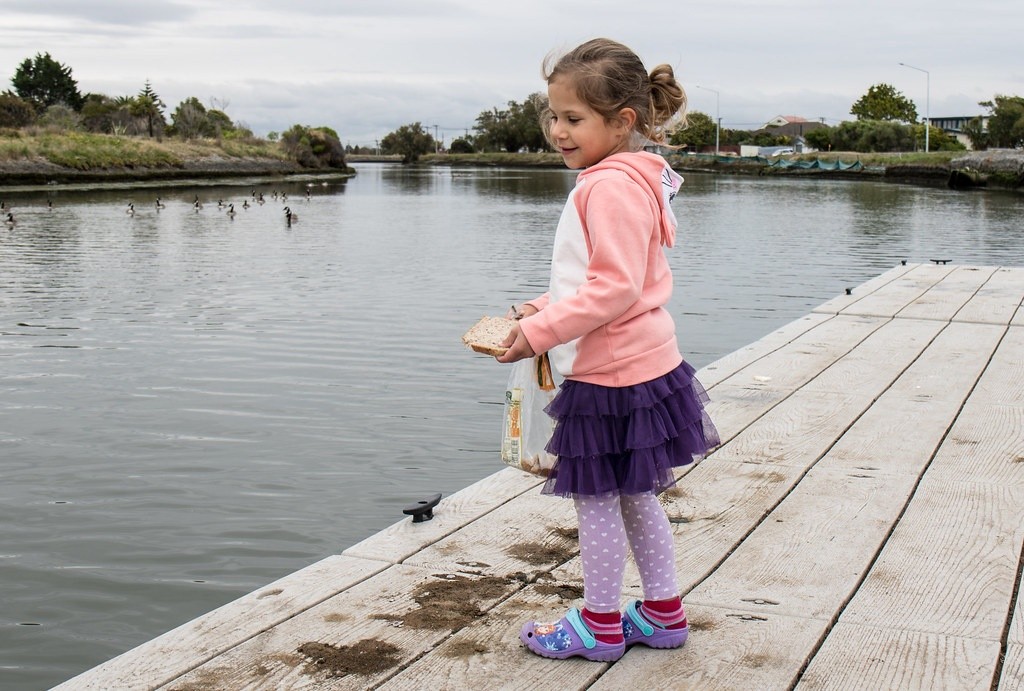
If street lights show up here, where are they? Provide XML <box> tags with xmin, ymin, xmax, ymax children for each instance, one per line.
<box><xmin>898</xmin><ymin>62</ymin><xmax>930</xmax><ymax>153</ymax></box>
<box><xmin>694</xmin><ymin>85</ymin><xmax>720</xmax><ymax>156</ymax></box>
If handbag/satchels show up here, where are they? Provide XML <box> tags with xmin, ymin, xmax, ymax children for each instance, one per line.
<box><xmin>501</xmin><ymin>304</ymin><xmax>563</xmax><ymax>480</ymax></box>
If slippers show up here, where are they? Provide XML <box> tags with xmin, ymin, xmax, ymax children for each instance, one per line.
<box><xmin>520</xmin><ymin>609</ymin><xmax>627</xmax><ymax>662</ymax></box>
<box><xmin>617</xmin><ymin>599</ymin><xmax>688</xmax><ymax>648</ymax></box>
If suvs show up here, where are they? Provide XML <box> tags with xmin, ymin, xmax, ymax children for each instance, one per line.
<box><xmin>772</xmin><ymin>149</ymin><xmax>794</xmax><ymax>157</ymax></box>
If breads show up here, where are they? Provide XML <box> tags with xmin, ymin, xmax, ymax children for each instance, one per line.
<box><xmin>460</xmin><ymin>316</ymin><xmax>521</xmax><ymax>355</ymax></box>
<box><xmin>522</xmin><ymin>451</ymin><xmax>557</xmax><ymax>477</ymax></box>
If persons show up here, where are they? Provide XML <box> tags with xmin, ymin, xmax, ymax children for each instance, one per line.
<box><xmin>493</xmin><ymin>37</ymin><xmax>721</xmax><ymax>665</ymax></box>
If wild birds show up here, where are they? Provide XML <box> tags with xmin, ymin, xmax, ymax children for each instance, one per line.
<box><xmin>0</xmin><ymin>189</ymin><xmax>312</xmax><ymax>227</ymax></box>
